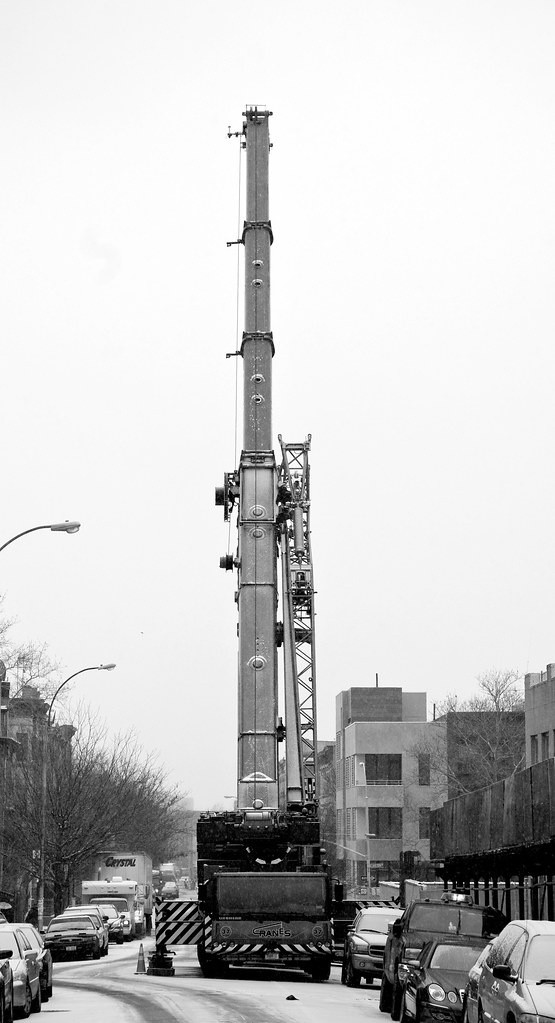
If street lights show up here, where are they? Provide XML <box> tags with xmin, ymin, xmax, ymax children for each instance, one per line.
<box><xmin>359</xmin><ymin>762</ymin><xmax>371</xmax><ymax>887</ymax></box>
<box><xmin>38</xmin><ymin>663</ymin><xmax>116</xmax><ymax>932</ymax></box>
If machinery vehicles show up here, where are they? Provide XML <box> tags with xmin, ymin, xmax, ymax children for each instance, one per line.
<box><xmin>148</xmin><ymin>104</ymin><xmax>401</xmax><ymax>982</ymax></box>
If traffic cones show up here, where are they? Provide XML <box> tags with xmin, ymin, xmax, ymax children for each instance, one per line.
<box><xmin>133</xmin><ymin>943</ymin><xmax>147</xmax><ymax>974</ymax></box>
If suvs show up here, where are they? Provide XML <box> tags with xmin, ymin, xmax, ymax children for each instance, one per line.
<box><xmin>341</xmin><ymin>892</ymin><xmax>555</xmax><ymax>1023</ymax></box>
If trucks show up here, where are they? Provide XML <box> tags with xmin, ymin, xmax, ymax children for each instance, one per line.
<box><xmin>81</xmin><ymin>850</ymin><xmax>180</xmax><ymax>942</ymax></box>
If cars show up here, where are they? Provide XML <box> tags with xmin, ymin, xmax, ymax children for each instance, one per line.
<box><xmin>39</xmin><ymin>904</ymin><xmax>131</xmax><ymax>960</ymax></box>
<box><xmin>0</xmin><ymin>913</ymin><xmax>53</xmax><ymax>1023</ymax></box>
